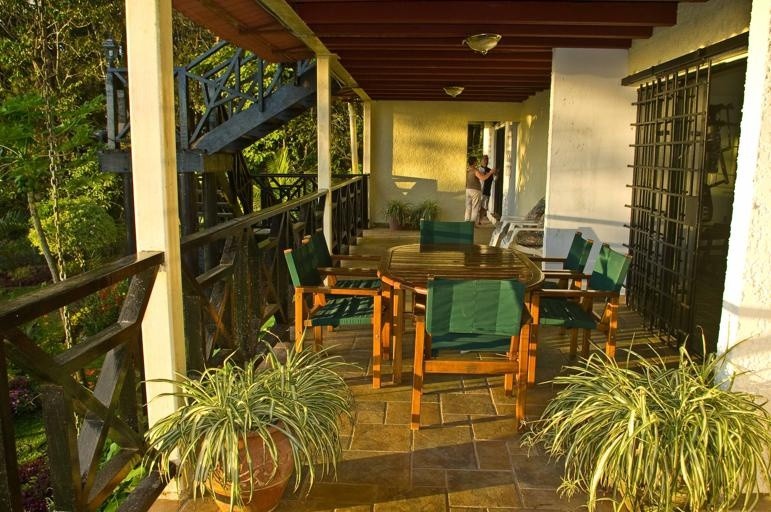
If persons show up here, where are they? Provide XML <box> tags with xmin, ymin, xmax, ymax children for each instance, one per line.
<box><xmin>476</xmin><ymin>155</ymin><xmax>495</xmax><ymax>224</ymax></box>
<box><xmin>465</xmin><ymin>155</ymin><xmax>497</xmax><ymax>224</ymax></box>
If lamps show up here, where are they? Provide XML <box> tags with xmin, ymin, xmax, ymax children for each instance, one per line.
<box><xmin>461</xmin><ymin>32</ymin><xmax>503</xmax><ymax>57</ymax></box>
<box><xmin>442</xmin><ymin>86</ymin><xmax>465</xmax><ymax>99</ymax></box>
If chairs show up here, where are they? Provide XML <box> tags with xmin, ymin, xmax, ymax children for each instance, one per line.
<box><xmin>490</xmin><ymin>194</ymin><xmax>545</xmax><ymax>256</ymax></box>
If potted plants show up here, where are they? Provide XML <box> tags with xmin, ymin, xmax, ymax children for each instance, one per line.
<box><xmin>136</xmin><ymin>313</ymin><xmax>365</xmax><ymax>511</ymax></box>
<box><xmin>383</xmin><ymin>199</ymin><xmax>442</xmax><ymax>231</ymax></box>
<box><xmin>519</xmin><ymin>324</ymin><xmax>771</xmax><ymax>511</ymax></box>
